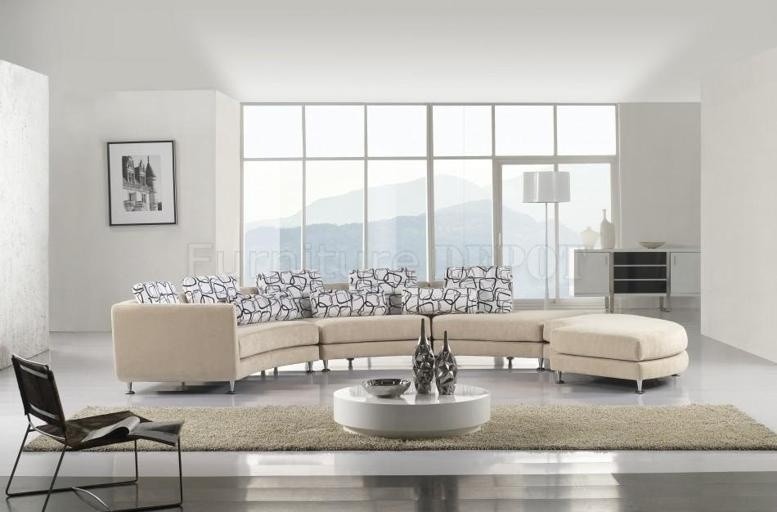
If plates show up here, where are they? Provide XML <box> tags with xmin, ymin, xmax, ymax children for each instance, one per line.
<box><xmin>573</xmin><ymin>249</ymin><xmax>671</xmax><ymax>313</ymax></box>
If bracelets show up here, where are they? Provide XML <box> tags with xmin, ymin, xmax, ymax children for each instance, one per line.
<box><xmin>362</xmin><ymin>378</ymin><xmax>411</xmax><ymax>397</ymax></box>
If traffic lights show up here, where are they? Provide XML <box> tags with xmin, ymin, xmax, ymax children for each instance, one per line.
<box><xmin>348</xmin><ymin>268</ymin><xmax>417</xmax><ymax>314</ymax></box>
<box><xmin>444</xmin><ymin>265</ymin><xmax>513</xmax><ymax>313</ymax></box>
<box><xmin>132</xmin><ymin>280</ymin><xmax>179</xmax><ymax>304</ymax></box>
<box><xmin>256</xmin><ymin>270</ymin><xmax>325</xmax><ymax>318</ymax></box>
<box><xmin>401</xmin><ymin>288</ymin><xmax>476</xmax><ymax>315</ymax></box>
<box><xmin>227</xmin><ymin>291</ymin><xmax>304</xmax><ymax>325</ymax></box>
<box><xmin>310</xmin><ymin>290</ymin><xmax>388</xmax><ymax>318</ymax></box>
<box><xmin>182</xmin><ymin>272</ymin><xmax>240</xmax><ymax>303</ymax></box>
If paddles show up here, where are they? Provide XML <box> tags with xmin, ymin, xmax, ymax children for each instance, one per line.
<box><xmin>23</xmin><ymin>404</ymin><xmax>777</xmax><ymax>449</ymax></box>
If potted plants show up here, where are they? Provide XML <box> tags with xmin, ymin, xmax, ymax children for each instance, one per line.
<box><xmin>107</xmin><ymin>140</ymin><xmax>176</xmax><ymax>225</ymax></box>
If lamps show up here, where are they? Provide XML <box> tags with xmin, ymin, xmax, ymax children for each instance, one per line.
<box><xmin>6</xmin><ymin>353</ymin><xmax>183</xmax><ymax>512</ymax></box>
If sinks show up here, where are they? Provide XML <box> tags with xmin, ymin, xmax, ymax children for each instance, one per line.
<box><xmin>111</xmin><ymin>282</ymin><xmax>689</xmax><ymax>394</ymax></box>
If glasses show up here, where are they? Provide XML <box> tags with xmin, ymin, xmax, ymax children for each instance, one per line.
<box><xmin>522</xmin><ymin>170</ymin><xmax>570</xmax><ymax>309</ymax></box>
